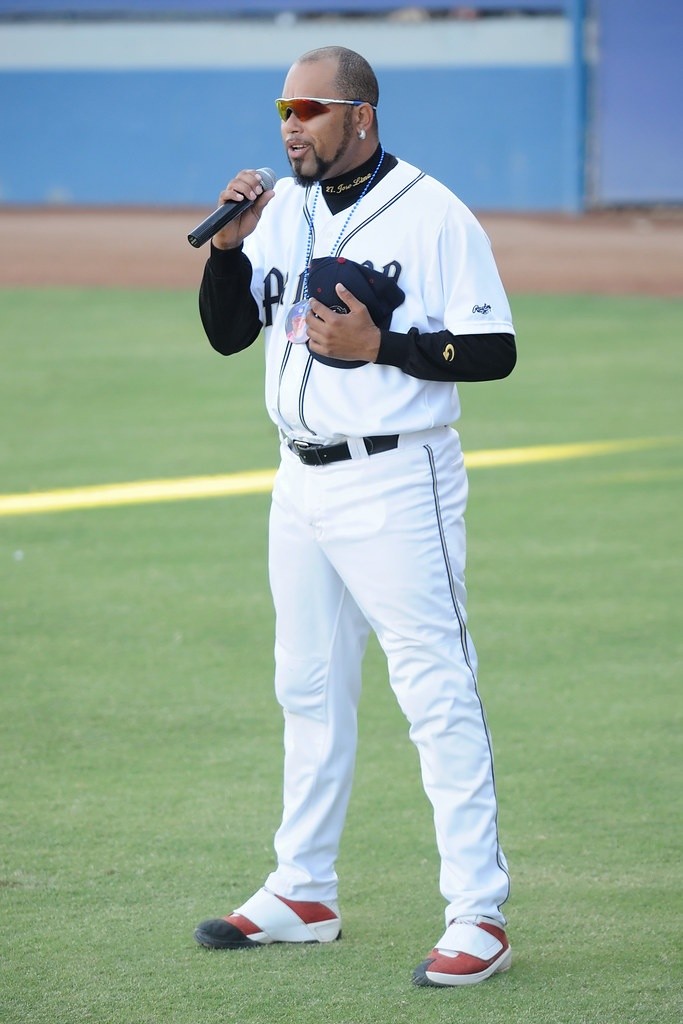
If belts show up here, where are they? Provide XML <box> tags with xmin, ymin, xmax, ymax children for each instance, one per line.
<box><xmin>284</xmin><ymin>435</ymin><xmax>399</xmax><ymax>467</ymax></box>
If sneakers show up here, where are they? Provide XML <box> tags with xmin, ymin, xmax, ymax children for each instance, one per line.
<box><xmin>196</xmin><ymin>888</ymin><xmax>346</xmax><ymax>950</ymax></box>
<box><xmin>414</xmin><ymin>920</ymin><xmax>515</xmax><ymax>987</ymax></box>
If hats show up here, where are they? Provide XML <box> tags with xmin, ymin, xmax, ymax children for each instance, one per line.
<box><xmin>306</xmin><ymin>258</ymin><xmax>405</xmax><ymax>370</ymax></box>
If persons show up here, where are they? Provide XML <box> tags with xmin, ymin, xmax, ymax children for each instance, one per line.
<box><xmin>194</xmin><ymin>45</ymin><xmax>517</xmax><ymax>988</ymax></box>
<box><xmin>287</xmin><ymin>305</ymin><xmax>308</xmax><ymax>343</ymax></box>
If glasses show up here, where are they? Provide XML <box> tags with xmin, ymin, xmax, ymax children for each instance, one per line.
<box><xmin>274</xmin><ymin>96</ymin><xmax>378</xmax><ymax>122</ymax></box>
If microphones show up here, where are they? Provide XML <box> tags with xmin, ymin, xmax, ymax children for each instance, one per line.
<box><xmin>188</xmin><ymin>167</ymin><xmax>277</xmax><ymax>249</ymax></box>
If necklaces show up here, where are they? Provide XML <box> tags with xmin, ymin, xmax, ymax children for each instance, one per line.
<box><xmin>284</xmin><ymin>144</ymin><xmax>384</xmax><ymax>343</ymax></box>
